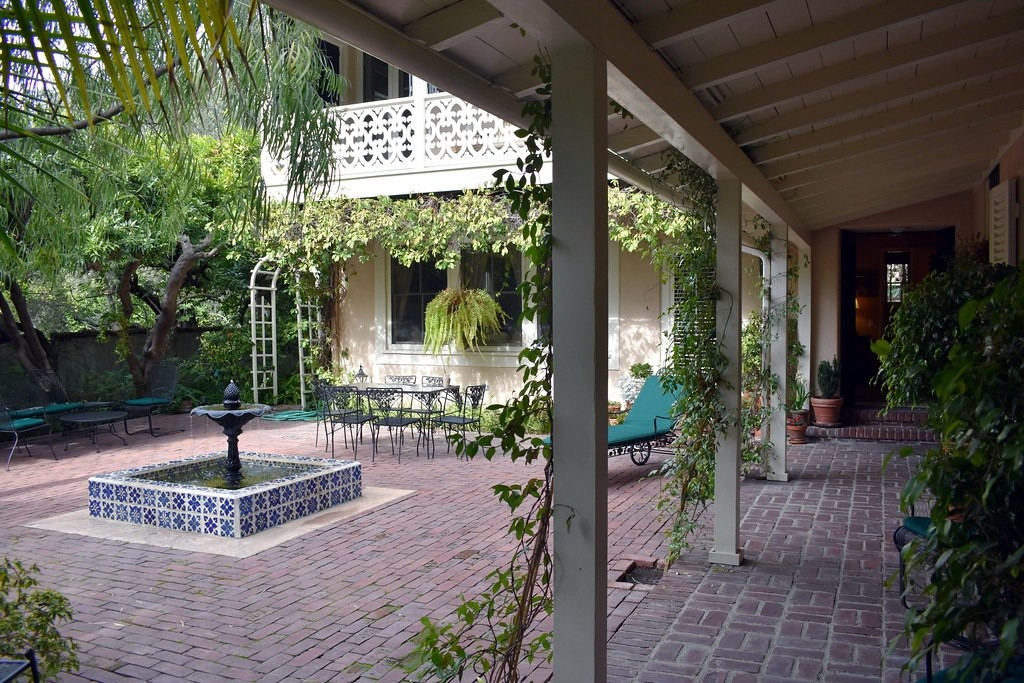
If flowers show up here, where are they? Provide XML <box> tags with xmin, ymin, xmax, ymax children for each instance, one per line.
<box><xmin>787</xmin><ymin>416</ymin><xmax>806</xmax><ymax>426</ymax></box>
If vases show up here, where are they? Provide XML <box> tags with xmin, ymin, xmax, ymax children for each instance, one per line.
<box><xmin>787</xmin><ymin>424</ymin><xmax>809</xmax><ymax>443</ymax></box>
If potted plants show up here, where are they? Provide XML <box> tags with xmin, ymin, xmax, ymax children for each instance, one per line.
<box><xmin>790</xmin><ymin>382</ymin><xmax>812</xmax><ymax>422</ymax></box>
<box><xmin>809</xmin><ymin>355</ymin><xmax>845</xmax><ymax>428</ymax></box>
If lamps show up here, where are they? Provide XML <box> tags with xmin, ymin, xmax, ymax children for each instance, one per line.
<box><xmin>355</xmin><ymin>365</ymin><xmax>367</xmax><ymax>383</ymax></box>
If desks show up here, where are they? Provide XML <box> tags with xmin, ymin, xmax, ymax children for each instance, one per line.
<box><xmin>325</xmin><ymin>383</ymin><xmax>460</xmax><ymax>460</ymax></box>
<box><xmin>60</xmin><ymin>410</ymin><xmax>128</xmax><ymax>453</ymax></box>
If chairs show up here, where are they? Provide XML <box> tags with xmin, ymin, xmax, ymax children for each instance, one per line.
<box><xmin>893</xmin><ymin>490</ymin><xmax>1004</xmax><ymax>651</ymax></box>
<box><xmin>365</xmin><ymin>387</ymin><xmax>436</xmax><ymax>463</ymax></box>
<box><xmin>540</xmin><ymin>374</ymin><xmax>690</xmax><ymax>465</ymax></box>
<box><xmin>372</xmin><ymin>375</ymin><xmax>418</xmax><ymax>446</ymax></box>
<box><xmin>310</xmin><ymin>378</ymin><xmax>364</xmax><ymax>450</ymax></box>
<box><xmin>402</xmin><ymin>376</ymin><xmax>450</xmax><ymax>459</ymax></box>
<box><xmin>0</xmin><ymin>397</ymin><xmax>59</xmax><ymax>472</ymax></box>
<box><xmin>324</xmin><ymin>386</ymin><xmax>380</xmax><ymax>460</ymax></box>
<box><xmin>0</xmin><ymin>400</ymin><xmax>118</xmax><ymax>457</ymax></box>
<box><xmin>124</xmin><ymin>360</ymin><xmax>185</xmax><ymax>439</ymax></box>
<box><xmin>430</xmin><ymin>384</ymin><xmax>486</xmax><ymax>461</ymax></box>
